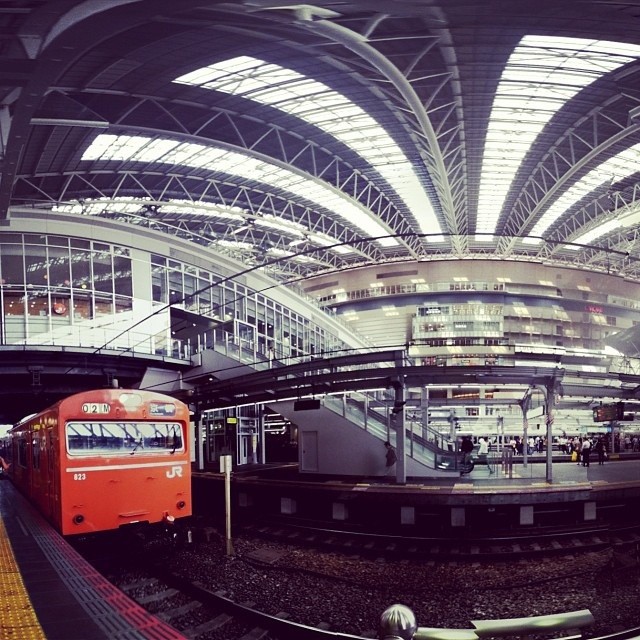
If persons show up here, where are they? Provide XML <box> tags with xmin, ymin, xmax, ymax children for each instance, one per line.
<box><xmin>620</xmin><ymin>437</ymin><xmax>640</xmax><ymax>452</ymax></box>
<box><xmin>596</xmin><ymin>438</ymin><xmax>607</xmax><ymax>465</ymax></box>
<box><xmin>382</xmin><ymin>441</ymin><xmax>396</xmax><ymax>486</ymax></box>
<box><xmin>581</xmin><ymin>438</ymin><xmax>591</xmax><ymax>468</ymax></box>
<box><xmin>459</xmin><ymin>436</ymin><xmax>474</xmax><ymax>462</ymax></box>
<box><xmin>557</xmin><ymin>437</ymin><xmax>582</xmax><ymax>465</ymax></box>
<box><xmin>510</xmin><ymin>436</ymin><xmax>543</xmax><ymax>456</ymax></box>
<box><xmin>472</xmin><ymin>436</ymin><xmax>495</xmax><ymax>474</ymax></box>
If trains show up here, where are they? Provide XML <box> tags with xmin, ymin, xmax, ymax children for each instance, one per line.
<box><xmin>1</xmin><ymin>388</ymin><xmax>196</xmax><ymax>557</ymax></box>
